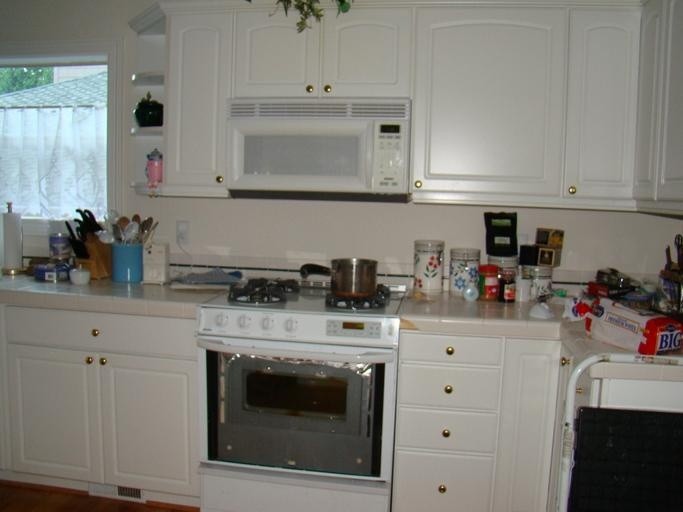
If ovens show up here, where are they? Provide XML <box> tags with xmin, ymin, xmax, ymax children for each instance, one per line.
<box><xmin>195</xmin><ymin>333</ymin><xmax>397</xmax><ymax>496</ymax></box>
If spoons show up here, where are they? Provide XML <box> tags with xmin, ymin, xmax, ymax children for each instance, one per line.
<box><xmin>111</xmin><ymin>215</ymin><xmax>158</xmax><ymax>245</ymax></box>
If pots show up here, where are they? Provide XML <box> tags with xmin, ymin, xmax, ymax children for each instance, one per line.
<box><xmin>299</xmin><ymin>258</ymin><xmax>378</xmax><ymax>297</ymax></box>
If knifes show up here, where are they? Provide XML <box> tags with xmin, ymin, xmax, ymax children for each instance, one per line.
<box><xmin>64</xmin><ymin>209</ymin><xmax>101</xmax><ymax>260</ymax></box>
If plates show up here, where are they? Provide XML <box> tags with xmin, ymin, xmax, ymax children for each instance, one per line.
<box><xmin>22</xmin><ymin>217</ymin><xmax>75</xmax><ymax>257</ymax></box>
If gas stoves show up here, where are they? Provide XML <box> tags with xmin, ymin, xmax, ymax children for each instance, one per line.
<box><xmin>227</xmin><ymin>277</ymin><xmax>390</xmax><ymax>309</ymax></box>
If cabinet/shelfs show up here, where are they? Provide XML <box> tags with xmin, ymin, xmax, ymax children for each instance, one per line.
<box><xmin>544</xmin><ymin>342</ymin><xmax>682</xmax><ymax>511</ymax></box>
<box><xmin>127</xmin><ymin>2</ymin><xmax>233</xmax><ymax>198</ymax></box>
<box><xmin>387</xmin><ymin>330</ymin><xmax>561</xmax><ymax>512</ymax></box>
<box><xmin>561</xmin><ymin>8</ymin><xmax>642</xmax><ymax>212</ymax></box>
<box><xmin>3</xmin><ymin>306</ymin><xmax>199</xmax><ymax>507</ymax></box>
<box><xmin>234</xmin><ymin>6</ymin><xmax>413</xmax><ymax>104</ymax></box>
<box><xmin>409</xmin><ymin>5</ymin><xmax>567</xmax><ymax>210</ymax></box>
<box><xmin>633</xmin><ymin>1</ymin><xmax>682</xmax><ymax>218</ymax></box>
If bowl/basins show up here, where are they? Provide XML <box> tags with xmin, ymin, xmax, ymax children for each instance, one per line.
<box><xmin>68</xmin><ymin>269</ymin><xmax>90</xmax><ymax>284</ymax></box>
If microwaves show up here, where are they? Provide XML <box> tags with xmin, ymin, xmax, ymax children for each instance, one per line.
<box><xmin>224</xmin><ymin>117</ymin><xmax>410</xmax><ymax>194</ymax></box>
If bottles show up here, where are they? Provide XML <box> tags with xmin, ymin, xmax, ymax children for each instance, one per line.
<box><xmin>145</xmin><ymin>149</ymin><xmax>162</xmax><ymax>184</ymax></box>
<box><xmin>414</xmin><ymin>239</ymin><xmax>552</xmax><ymax>304</ymax></box>
<box><xmin>1</xmin><ymin>202</ymin><xmax>23</xmax><ymax>275</ymax></box>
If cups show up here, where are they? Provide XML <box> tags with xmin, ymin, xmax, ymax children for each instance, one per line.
<box><xmin>111</xmin><ymin>243</ymin><xmax>142</xmax><ymax>283</ymax></box>
